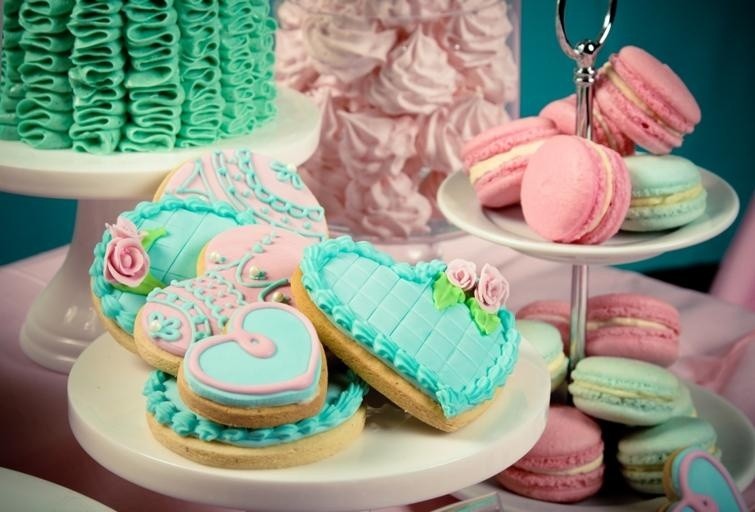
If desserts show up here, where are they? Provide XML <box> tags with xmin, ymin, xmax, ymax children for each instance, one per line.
<box><xmin>1</xmin><ymin>1</ymin><xmax>755</xmax><ymax>512</ymax></box>
